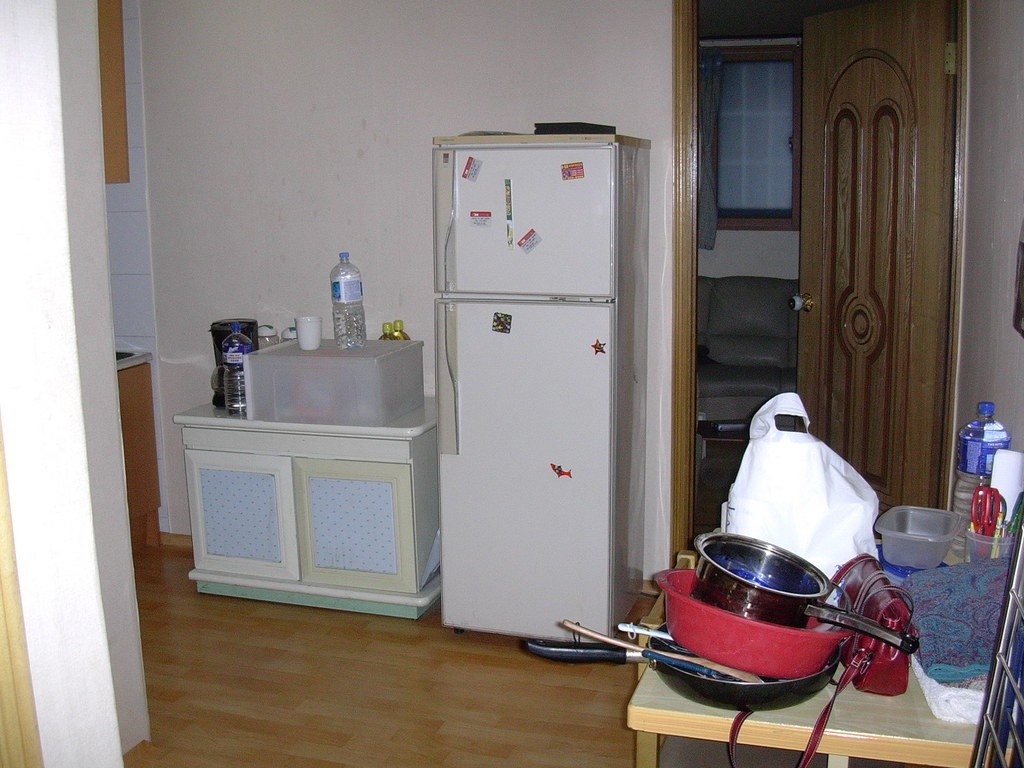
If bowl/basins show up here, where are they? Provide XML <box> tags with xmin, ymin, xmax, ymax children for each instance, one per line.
<box><xmin>964</xmin><ymin>524</ymin><xmax>1015</xmax><ymax>562</ymax></box>
<box><xmin>874</xmin><ymin>505</ymin><xmax>962</xmax><ymax>570</ymax></box>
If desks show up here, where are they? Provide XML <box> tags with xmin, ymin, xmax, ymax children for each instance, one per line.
<box><xmin>627</xmin><ymin>662</ymin><xmax>1014</xmax><ymax>768</ymax></box>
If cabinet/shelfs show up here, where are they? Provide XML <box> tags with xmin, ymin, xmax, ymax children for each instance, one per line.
<box><xmin>171</xmin><ymin>400</ymin><xmax>441</xmax><ymax>621</ymax></box>
<box><xmin>117</xmin><ymin>363</ymin><xmax>163</xmax><ymax>567</ymax></box>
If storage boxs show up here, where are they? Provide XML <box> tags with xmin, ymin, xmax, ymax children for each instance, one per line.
<box><xmin>242</xmin><ymin>338</ymin><xmax>425</xmax><ymax>427</ymax></box>
<box><xmin>874</xmin><ymin>504</ymin><xmax>967</xmax><ymax>584</ymax></box>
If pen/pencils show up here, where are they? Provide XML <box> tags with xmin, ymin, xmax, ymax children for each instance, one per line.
<box><xmin>971</xmin><ymin>492</ymin><xmax>1024</xmax><ymax>559</ymax></box>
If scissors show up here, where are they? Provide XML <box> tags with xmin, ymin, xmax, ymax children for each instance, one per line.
<box><xmin>971</xmin><ymin>486</ymin><xmax>1007</xmax><ymax>536</ymax></box>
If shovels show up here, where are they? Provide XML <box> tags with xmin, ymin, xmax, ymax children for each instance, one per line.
<box><xmin>561</xmin><ymin>619</ymin><xmax>767</xmax><ymax>683</ymax></box>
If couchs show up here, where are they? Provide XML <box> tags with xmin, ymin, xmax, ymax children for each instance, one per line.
<box><xmin>695</xmin><ymin>275</ymin><xmax>800</xmax><ymax>421</ymax></box>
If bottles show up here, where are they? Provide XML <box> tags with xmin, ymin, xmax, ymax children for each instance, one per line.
<box><xmin>221</xmin><ymin>323</ymin><xmax>256</xmax><ymax>420</ymax></box>
<box><xmin>257</xmin><ymin>324</ymin><xmax>298</xmax><ymax>350</ymax></box>
<box><xmin>330</xmin><ymin>252</ymin><xmax>367</xmax><ymax>353</ymax></box>
<box><xmin>377</xmin><ymin>320</ymin><xmax>410</xmax><ymax>341</ymax></box>
<box><xmin>951</xmin><ymin>402</ymin><xmax>1012</xmax><ymax>537</ymax></box>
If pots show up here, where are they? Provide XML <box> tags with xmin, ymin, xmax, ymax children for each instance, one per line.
<box><xmin>522</xmin><ymin>530</ymin><xmax>920</xmax><ymax>713</ymax></box>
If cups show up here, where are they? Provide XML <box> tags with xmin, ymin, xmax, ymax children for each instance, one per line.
<box><xmin>294</xmin><ymin>316</ymin><xmax>323</xmax><ymax>351</ymax></box>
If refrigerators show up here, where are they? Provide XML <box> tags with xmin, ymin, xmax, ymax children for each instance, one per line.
<box><xmin>430</xmin><ymin>132</ymin><xmax>653</xmax><ymax>647</ymax></box>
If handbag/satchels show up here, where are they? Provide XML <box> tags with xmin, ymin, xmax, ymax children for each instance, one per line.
<box><xmin>725</xmin><ymin>391</ymin><xmax>880</xmax><ymax>604</ymax></box>
<box><xmin>837</xmin><ymin>553</ymin><xmax>919</xmax><ymax>696</ymax></box>
